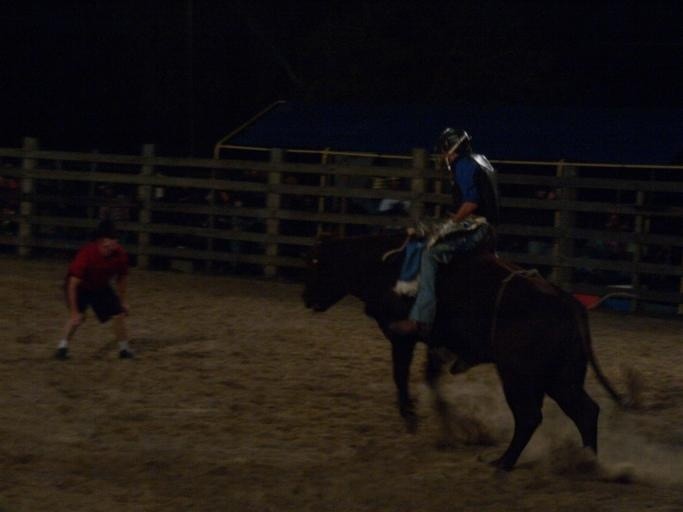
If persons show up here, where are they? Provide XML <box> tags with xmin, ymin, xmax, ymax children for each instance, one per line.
<box><xmin>54</xmin><ymin>220</ymin><xmax>135</xmax><ymax>360</ymax></box>
<box><xmin>390</xmin><ymin>127</ymin><xmax>499</xmax><ymax>336</ymax></box>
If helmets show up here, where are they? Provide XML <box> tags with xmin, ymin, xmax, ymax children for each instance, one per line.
<box><xmin>439</xmin><ymin>127</ymin><xmax>473</xmax><ymax>158</ymax></box>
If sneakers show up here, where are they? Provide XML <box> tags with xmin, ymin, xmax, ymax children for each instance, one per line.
<box><xmin>56</xmin><ymin>347</ymin><xmax>71</xmax><ymax>362</ymax></box>
<box><xmin>390</xmin><ymin>320</ymin><xmax>431</xmax><ymax>334</ymax></box>
<box><xmin>119</xmin><ymin>349</ymin><xmax>133</xmax><ymax>358</ymax></box>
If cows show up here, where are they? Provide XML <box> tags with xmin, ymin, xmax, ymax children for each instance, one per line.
<box><xmin>300</xmin><ymin>229</ymin><xmax>640</xmax><ymax>472</ymax></box>
